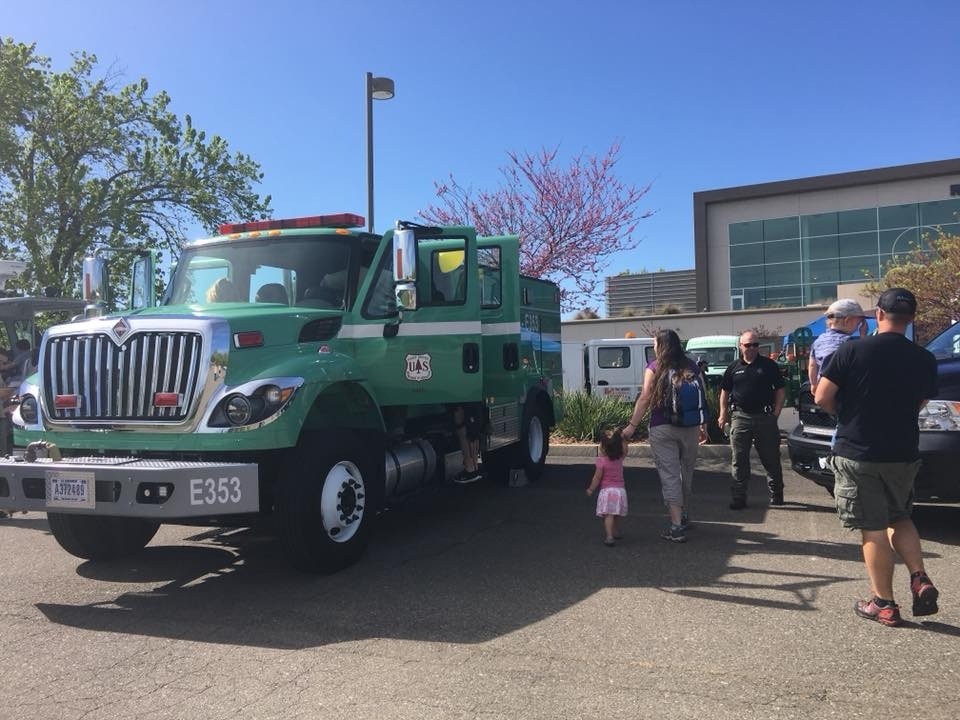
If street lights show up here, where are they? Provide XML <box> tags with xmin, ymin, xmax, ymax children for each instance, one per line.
<box><xmin>366</xmin><ymin>69</ymin><xmax>395</xmax><ymax>236</ymax></box>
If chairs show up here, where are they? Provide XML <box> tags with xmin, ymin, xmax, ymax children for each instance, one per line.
<box><xmin>256</xmin><ymin>283</ymin><xmax>289</xmax><ymax>305</ymax></box>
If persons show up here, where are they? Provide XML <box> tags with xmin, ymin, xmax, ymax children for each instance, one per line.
<box><xmin>0</xmin><ymin>338</ymin><xmax>34</xmax><ymax>382</ymax></box>
<box><xmin>812</xmin><ymin>287</ymin><xmax>943</xmax><ymax>627</ymax></box>
<box><xmin>717</xmin><ymin>327</ymin><xmax>787</xmax><ymax>511</ymax></box>
<box><xmin>808</xmin><ymin>298</ymin><xmax>870</xmax><ymax>396</ymax></box>
<box><xmin>451</xmin><ymin>403</ymin><xmax>484</xmax><ymax>485</ymax></box>
<box><xmin>205</xmin><ymin>276</ymin><xmax>241</xmax><ymax>302</ymax></box>
<box><xmin>320</xmin><ymin>232</ymin><xmax>394</xmax><ymax>315</ymax></box>
<box><xmin>585</xmin><ymin>423</ymin><xmax>629</xmax><ymax>546</ymax></box>
<box><xmin>620</xmin><ymin>328</ymin><xmax>713</xmax><ymax>544</ymax></box>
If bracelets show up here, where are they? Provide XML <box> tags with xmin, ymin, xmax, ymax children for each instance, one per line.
<box><xmin>629</xmin><ymin>420</ymin><xmax>642</xmax><ymax>427</ymax></box>
<box><xmin>588</xmin><ymin>487</ymin><xmax>594</xmax><ymax>492</ymax></box>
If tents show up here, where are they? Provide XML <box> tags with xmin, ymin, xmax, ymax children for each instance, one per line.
<box><xmin>782</xmin><ymin>313</ymin><xmax>879</xmax><ymax>386</ymax></box>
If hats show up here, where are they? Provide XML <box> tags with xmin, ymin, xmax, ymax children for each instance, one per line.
<box><xmin>877</xmin><ymin>287</ymin><xmax>916</xmax><ymax>313</ymax></box>
<box><xmin>827</xmin><ymin>299</ymin><xmax>876</xmax><ymax>319</ymax></box>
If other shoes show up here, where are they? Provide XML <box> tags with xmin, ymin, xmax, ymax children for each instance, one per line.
<box><xmin>603</xmin><ymin>539</ymin><xmax>615</xmax><ymax>545</ymax></box>
<box><xmin>729</xmin><ymin>494</ymin><xmax>746</xmax><ymax>509</ymax></box>
<box><xmin>614</xmin><ymin>534</ymin><xmax>619</xmax><ymax>538</ymax></box>
<box><xmin>769</xmin><ymin>487</ymin><xmax>783</xmax><ymax>504</ymax></box>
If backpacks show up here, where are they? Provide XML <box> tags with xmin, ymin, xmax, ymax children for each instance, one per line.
<box><xmin>660</xmin><ymin>364</ymin><xmax>709</xmax><ymax>426</ymax></box>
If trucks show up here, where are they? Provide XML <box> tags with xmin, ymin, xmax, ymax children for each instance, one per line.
<box><xmin>561</xmin><ymin>337</ymin><xmax>652</xmax><ymax>406</ymax></box>
<box><xmin>685</xmin><ymin>334</ymin><xmax>775</xmax><ymax>386</ymax></box>
<box><xmin>0</xmin><ymin>211</ymin><xmax>563</xmax><ymax>582</ymax></box>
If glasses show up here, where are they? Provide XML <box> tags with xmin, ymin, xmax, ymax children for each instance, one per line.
<box><xmin>741</xmin><ymin>343</ymin><xmax>759</xmax><ymax>348</ymax></box>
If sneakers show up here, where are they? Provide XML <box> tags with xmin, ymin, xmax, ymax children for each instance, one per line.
<box><xmin>661</xmin><ymin>521</ymin><xmax>686</xmax><ymax>542</ymax></box>
<box><xmin>855</xmin><ymin>599</ymin><xmax>901</xmax><ymax>625</ymax></box>
<box><xmin>911</xmin><ymin>576</ymin><xmax>938</xmax><ymax>616</ymax></box>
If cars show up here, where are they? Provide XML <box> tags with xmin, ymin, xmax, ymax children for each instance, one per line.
<box><xmin>787</xmin><ymin>319</ymin><xmax>959</xmax><ymax>512</ymax></box>
